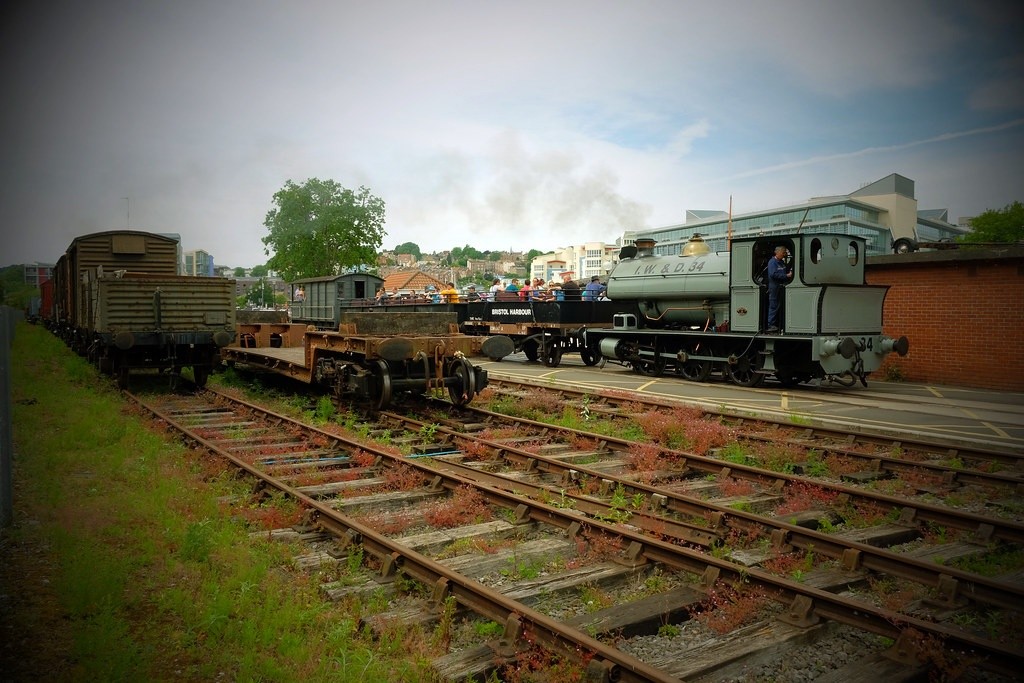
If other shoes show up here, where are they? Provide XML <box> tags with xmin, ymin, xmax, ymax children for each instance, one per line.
<box><xmin>768</xmin><ymin>326</ymin><xmax>780</xmax><ymax>331</ymax></box>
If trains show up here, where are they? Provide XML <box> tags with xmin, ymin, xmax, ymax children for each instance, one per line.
<box><xmin>296</xmin><ymin>201</ymin><xmax>911</xmax><ymax>387</ymax></box>
<box><xmin>29</xmin><ymin>227</ymin><xmax>237</xmax><ymax>396</ymax></box>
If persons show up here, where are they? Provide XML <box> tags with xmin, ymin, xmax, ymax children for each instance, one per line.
<box><xmin>375</xmin><ymin>273</ymin><xmax>607</xmax><ymax>302</ymax></box>
<box><xmin>766</xmin><ymin>246</ymin><xmax>792</xmax><ymax>331</ymax></box>
<box><xmin>296</xmin><ymin>286</ymin><xmax>307</xmax><ymax>302</ymax></box>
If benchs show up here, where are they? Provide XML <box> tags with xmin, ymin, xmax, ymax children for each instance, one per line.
<box><xmin>495</xmin><ymin>291</ymin><xmax>519</xmax><ymax>302</ymax></box>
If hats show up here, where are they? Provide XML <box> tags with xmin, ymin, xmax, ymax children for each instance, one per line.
<box><xmin>468</xmin><ymin>286</ymin><xmax>474</xmax><ymax>289</ymax></box>
<box><xmin>429</xmin><ymin>286</ymin><xmax>435</xmax><ymax>290</ymax></box>
<box><xmin>591</xmin><ymin>276</ymin><xmax>599</xmax><ymax>281</ymax></box>
